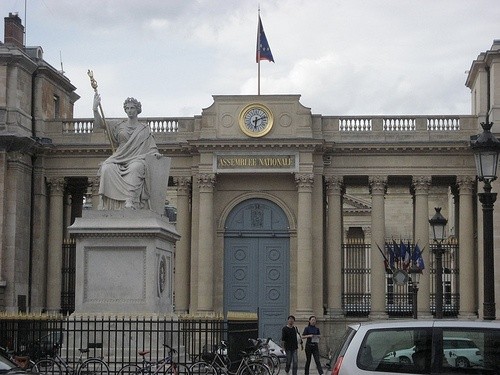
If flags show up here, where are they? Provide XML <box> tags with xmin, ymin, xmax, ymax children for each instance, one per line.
<box><xmin>256</xmin><ymin>15</ymin><xmax>275</xmax><ymax>63</ymax></box>
<box><xmin>375</xmin><ymin>238</ymin><xmax>425</xmax><ymax>273</ymax></box>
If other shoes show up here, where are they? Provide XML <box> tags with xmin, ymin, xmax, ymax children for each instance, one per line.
<box><xmin>285</xmin><ymin>372</ymin><xmax>290</xmax><ymax>375</ymax></box>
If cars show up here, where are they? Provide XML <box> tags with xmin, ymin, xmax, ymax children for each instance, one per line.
<box><xmin>0</xmin><ymin>346</ymin><xmax>26</xmax><ymax>375</ymax></box>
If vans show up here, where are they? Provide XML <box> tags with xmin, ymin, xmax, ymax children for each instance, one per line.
<box><xmin>322</xmin><ymin>317</ymin><xmax>500</xmax><ymax>375</ymax></box>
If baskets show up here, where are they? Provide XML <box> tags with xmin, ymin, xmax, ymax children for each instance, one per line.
<box><xmin>202</xmin><ymin>344</ymin><xmax>217</xmax><ymax>361</ymax></box>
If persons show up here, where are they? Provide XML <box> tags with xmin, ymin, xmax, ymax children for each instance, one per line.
<box><xmin>92</xmin><ymin>92</ymin><xmax>163</xmax><ymax>210</ymax></box>
<box><xmin>301</xmin><ymin>316</ymin><xmax>324</xmax><ymax>375</ymax></box>
<box><xmin>281</xmin><ymin>315</ymin><xmax>304</xmax><ymax>375</ymax></box>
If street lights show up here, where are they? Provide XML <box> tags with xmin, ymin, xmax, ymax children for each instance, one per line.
<box><xmin>467</xmin><ymin>121</ymin><xmax>500</xmax><ymax>320</ymax></box>
<box><xmin>428</xmin><ymin>207</ymin><xmax>448</xmax><ymax>319</ymax></box>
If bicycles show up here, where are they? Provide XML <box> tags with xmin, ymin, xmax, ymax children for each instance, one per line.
<box><xmin>5</xmin><ymin>336</ymin><xmax>283</xmax><ymax>375</ymax></box>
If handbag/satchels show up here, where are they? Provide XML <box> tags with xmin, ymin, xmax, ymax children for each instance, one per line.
<box><xmin>295</xmin><ymin>326</ymin><xmax>301</xmax><ymax>344</ymax></box>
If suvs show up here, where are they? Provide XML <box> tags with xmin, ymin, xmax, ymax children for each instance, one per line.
<box><xmin>383</xmin><ymin>336</ymin><xmax>484</xmax><ymax>369</ymax></box>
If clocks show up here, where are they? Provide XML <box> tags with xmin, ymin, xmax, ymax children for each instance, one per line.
<box><xmin>238</xmin><ymin>102</ymin><xmax>274</xmax><ymax>138</ymax></box>
